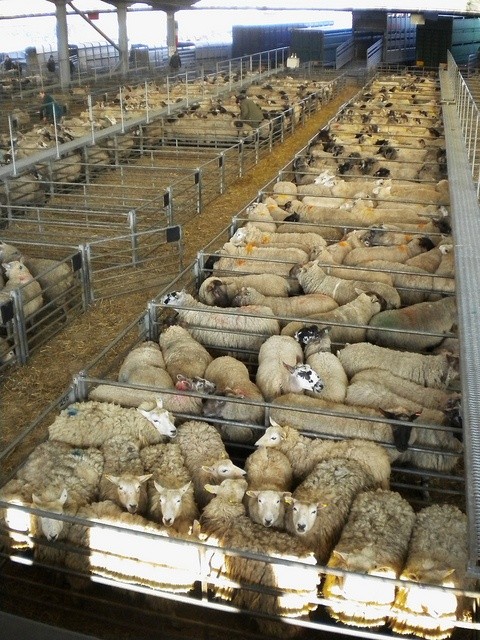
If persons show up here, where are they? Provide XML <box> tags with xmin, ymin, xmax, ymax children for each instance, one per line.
<box><xmin>46</xmin><ymin>55</ymin><xmax>56</xmax><ymax>72</ymax></box>
<box><xmin>69</xmin><ymin>60</ymin><xmax>76</xmax><ymax>81</ymax></box>
<box><xmin>235</xmin><ymin>95</ymin><xmax>264</xmax><ymax>128</ymax></box>
<box><xmin>3</xmin><ymin>55</ymin><xmax>12</xmax><ymax>70</ymax></box>
<box><xmin>169</xmin><ymin>51</ymin><xmax>181</xmax><ymax>82</ymax></box>
<box><xmin>39</xmin><ymin>89</ymin><xmax>62</xmax><ymax>124</ymax></box>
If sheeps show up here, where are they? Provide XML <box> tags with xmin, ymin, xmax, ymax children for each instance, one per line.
<box><xmin>366</xmin><ymin>296</ymin><xmax>458</xmax><ymax>348</ymax></box>
<box><xmin>389</xmin><ymin>503</ymin><xmax>471</xmax><ymax>639</ymax></box>
<box><xmin>285</xmin><ymin>457</ymin><xmax>364</xmax><ymax>552</ymax></box>
<box><xmin>200</xmin><ymin>478</ymin><xmax>321</xmax><ymax>619</ymax></box>
<box><xmin>67</xmin><ymin>500</ymin><xmax>228</xmax><ymax>594</ymax></box>
<box><xmin>48</xmin><ymin>397</ymin><xmax>178</xmax><ymax>449</ymax></box>
<box><xmin>336</xmin><ymin>341</ymin><xmax>460</xmax><ymax>386</ymax></box>
<box><xmin>0</xmin><ymin>257</ymin><xmax>43</xmax><ymax>324</ymax></box>
<box><xmin>323</xmin><ymin>488</ymin><xmax>417</xmax><ymax>628</ymax></box>
<box><xmin>281</xmin><ymin>287</ymin><xmax>389</xmax><ymax>340</ymax></box>
<box><xmin>88</xmin><ymin>373</ymin><xmax>216</xmax><ymax>415</ymax></box>
<box><xmin>289</xmin><ymin>258</ymin><xmax>401</xmax><ymax>309</ymax></box>
<box><xmin>31</xmin><ymin>447</ymin><xmax>103</xmax><ymax>560</ymax></box>
<box><xmin>294</xmin><ymin>324</ymin><xmax>349</xmax><ymax>403</ymax></box>
<box><xmin>159</xmin><ymin>289</ymin><xmax>281</xmax><ymax>351</ymax></box>
<box><xmin>231</xmin><ymin>286</ymin><xmax>340</xmax><ymax>324</ymax></box>
<box><xmin>172</xmin><ymin>421</ymin><xmax>247</xmax><ymax>495</ymax></box>
<box><xmin>1</xmin><ymin>68</ymin><xmax>451</xmax><ymax>283</ymax></box>
<box><xmin>198</xmin><ymin>273</ymin><xmax>291</xmax><ymax>306</ymax></box>
<box><xmin>1</xmin><ymin>442</ymin><xmax>73</xmax><ymax>552</ymax></box>
<box><xmin>140</xmin><ymin>442</ymin><xmax>197</xmax><ymax>528</ymax></box>
<box><xmin>157</xmin><ymin>324</ymin><xmax>212</xmax><ymax>385</ymax></box>
<box><xmin>341</xmin><ymin>369</ymin><xmax>460</xmax><ymax>420</ymax></box>
<box><xmin>254</xmin><ymin>416</ymin><xmax>390</xmax><ymax>490</ymax></box>
<box><xmin>118</xmin><ymin>341</ymin><xmax>175</xmax><ymax>389</ymax></box>
<box><xmin>268</xmin><ymin>394</ymin><xmax>424</xmax><ymax>464</ymax></box>
<box><xmin>256</xmin><ymin>334</ymin><xmax>324</xmax><ymax>402</ymax></box>
<box><xmin>202</xmin><ymin>356</ymin><xmax>265</xmax><ymax>442</ymax></box>
<box><xmin>99</xmin><ymin>438</ymin><xmax>148</xmax><ymax>516</ymax></box>
<box><xmin>244</xmin><ymin>449</ymin><xmax>293</xmax><ymax>529</ymax></box>
